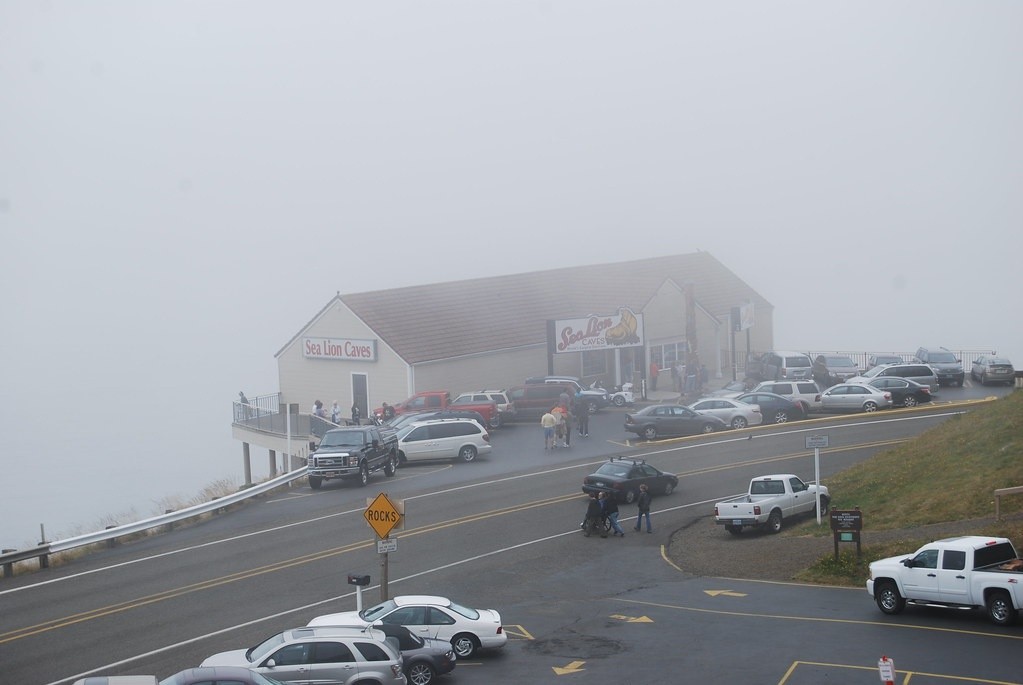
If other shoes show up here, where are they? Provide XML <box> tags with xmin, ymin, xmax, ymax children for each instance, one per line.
<box><xmin>647</xmin><ymin>529</ymin><xmax>652</xmax><ymax>533</ymax></box>
<box><xmin>545</xmin><ymin>426</ymin><xmax>589</xmax><ymax>449</ymax></box>
<box><xmin>621</xmin><ymin>532</ymin><xmax>624</xmax><ymax>536</ymax></box>
<box><xmin>600</xmin><ymin>535</ymin><xmax>607</xmax><ymax>538</ymax></box>
<box><xmin>613</xmin><ymin>530</ymin><xmax>617</xmax><ymax>534</ymax></box>
<box><xmin>584</xmin><ymin>533</ymin><xmax>590</xmax><ymax>537</ymax></box>
<box><xmin>634</xmin><ymin>526</ymin><xmax>640</xmax><ymax>532</ymax></box>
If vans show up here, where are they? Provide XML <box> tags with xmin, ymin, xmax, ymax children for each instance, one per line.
<box><xmin>751</xmin><ymin>350</ymin><xmax>815</xmax><ymax>384</ymax></box>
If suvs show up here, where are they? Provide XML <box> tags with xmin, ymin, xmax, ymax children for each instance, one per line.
<box><xmin>523</xmin><ymin>375</ymin><xmax>612</xmax><ymax>415</ymax></box>
<box><xmin>912</xmin><ymin>345</ymin><xmax>965</xmax><ymax>387</ymax></box>
<box><xmin>740</xmin><ymin>377</ymin><xmax>822</xmax><ymax>413</ymax></box>
<box><xmin>450</xmin><ymin>388</ymin><xmax>519</xmax><ymax>431</ymax></box>
<box><xmin>307</xmin><ymin>424</ymin><xmax>400</xmax><ymax>490</ymax></box>
<box><xmin>844</xmin><ymin>361</ymin><xmax>940</xmax><ymax>395</ymax></box>
<box><xmin>499</xmin><ymin>382</ymin><xmax>581</xmax><ymax>423</ymax></box>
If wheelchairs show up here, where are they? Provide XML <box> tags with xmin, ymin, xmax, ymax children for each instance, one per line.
<box><xmin>580</xmin><ymin>508</ymin><xmax>612</xmax><ymax>533</ymax></box>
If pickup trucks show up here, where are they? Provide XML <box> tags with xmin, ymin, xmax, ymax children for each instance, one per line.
<box><xmin>713</xmin><ymin>473</ymin><xmax>832</xmax><ymax>535</ymax></box>
<box><xmin>866</xmin><ymin>535</ymin><xmax>1023</xmax><ymax>627</ymax></box>
<box><xmin>379</xmin><ymin>391</ymin><xmax>499</xmax><ymax>431</ymax></box>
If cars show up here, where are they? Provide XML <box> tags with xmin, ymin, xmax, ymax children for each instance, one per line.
<box><xmin>374</xmin><ymin>408</ymin><xmax>492</xmax><ymax>470</ymax></box>
<box><xmin>367</xmin><ymin>623</ymin><xmax>458</xmax><ymax>685</ymax></box>
<box><xmin>580</xmin><ymin>455</ymin><xmax>679</xmax><ymax>504</ymax></box>
<box><xmin>970</xmin><ymin>355</ymin><xmax>1016</xmax><ymax>387</ymax></box>
<box><xmin>158</xmin><ymin>665</ymin><xmax>281</xmax><ymax>685</ymax></box>
<box><xmin>849</xmin><ymin>376</ymin><xmax>931</xmax><ymax>409</ymax></box>
<box><xmin>721</xmin><ymin>391</ymin><xmax>804</xmax><ymax>426</ymax></box>
<box><xmin>668</xmin><ymin>396</ymin><xmax>764</xmax><ymax>430</ymax></box>
<box><xmin>812</xmin><ymin>353</ymin><xmax>860</xmax><ymax>387</ymax></box>
<box><xmin>818</xmin><ymin>382</ymin><xmax>894</xmax><ymax>414</ymax></box>
<box><xmin>623</xmin><ymin>402</ymin><xmax>727</xmax><ymax>442</ymax></box>
<box><xmin>197</xmin><ymin>624</ymin><xmax>409</xmax><ymax>685</ymax></box>
<box><xmin>304</xmin><ymin>594</ymin><xmax>508</xmax><ymax>661</ymax></box>
<box><xmin>865</xmin><ymin>353</ymin><xmax>904</xmax><ymax>372</ymax></box>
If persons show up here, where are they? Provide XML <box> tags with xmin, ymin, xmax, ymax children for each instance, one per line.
<box><xmin>383</xmin><ymin>402</ymin><xmax>396</xmax><ymax>422</ymax></box>
<box><xmin>542</xmin><ymin>388</ymin><xmax>589</xmax><ymax>449</ymax></box>
<box><xmin>312</xmin><ymin>401</ymin><xmax>327</xmax><ymax>418</ymax></box>
<box><xmin>330</xmin><ymin>402</ymin><xmax>341</xmax><ymax>425</ymax></box>
<box><xmin>651</xmin><ymin>360</ymin><xmax>659</xmax><ymax>391</ymax></box>
<box><xmin>352</xmin><ymin>402</ymin><xmax>360</xmax><ymax>425</ymax></box>
<box><xmin>240</xmin><ymin>392</ymin><xmax>254</xmax><ymax>424</ymax></box>
<box><xmin>584</xmin><ymin>492</ymin><xmax>624</xmax><ymax>537</ymax></box>
<box><xmin>634</xmin><ymin>485</ymin><xmax>652</xmax><ymax>533</ymax></box>
<box><xmin>670</xmin><ymin>363</ymin><xmax>708</xmax><ymax>393</ymax></box>
